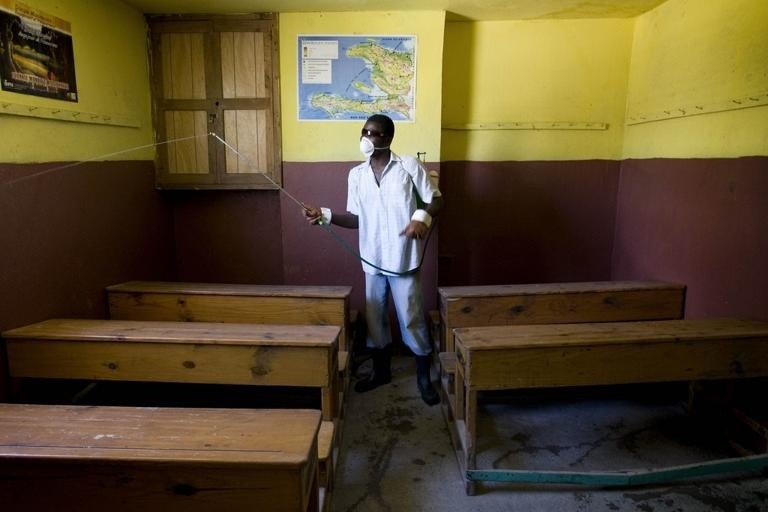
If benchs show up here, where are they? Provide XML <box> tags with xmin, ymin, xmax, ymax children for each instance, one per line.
<box><xmin>1</xmin><ymin>283</ymin><xmax>356</xmax><ymax>511</ymax></box>
<box><xmin>427</xmin><ymin>311</ymin><xmax>437</xmax><ymax>328</ymax></box>
<box><xmin>438</xmin><ymin>353</ymin><xmax>456</xmax><ymax>423</ymax></box>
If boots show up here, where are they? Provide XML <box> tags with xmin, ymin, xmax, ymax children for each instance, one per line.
<box><xmin>354</xmin><ymin>348</ymin><xmax>391</xmax><ymax>393</ymax></box>
<box><xmin>416</xmin><ymin>353</ymin><xmax>441</xmax><ymax>405</ymax></box>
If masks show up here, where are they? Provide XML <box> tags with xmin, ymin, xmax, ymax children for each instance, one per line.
<box><xmin>360</xmin><ymin>138</ymin><xmax>390</xmax><ymax>157</ymax></box>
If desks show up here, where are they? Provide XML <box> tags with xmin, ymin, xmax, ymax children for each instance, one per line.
<box><xmin>452</xmin><ymin>318</ymin><xmax>768</xmax><ymax>493</ymax></box>
<box><xmin>437</xmin><ymin>281</ymin><xmax>688</xmax><ymax>351</ymax></box>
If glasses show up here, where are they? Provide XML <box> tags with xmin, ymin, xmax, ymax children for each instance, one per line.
<box><xmin>362</xmin><ymin>128</ymin><xmax>381</xmax><ymax>137</ymax></box>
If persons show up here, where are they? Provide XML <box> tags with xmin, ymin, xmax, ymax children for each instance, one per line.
<box><xmin>301</xmin><ymin>114</ymin><xmax>443</xmax><ymax>406</ymax></box>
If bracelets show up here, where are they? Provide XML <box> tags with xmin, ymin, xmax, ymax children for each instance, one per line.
<box><xmin>410</xmin><ymin>209</ymin><xmax>432</xmax><ymax>229</ymax></box>
<box><xmin>319</xmin><ymin>206</ymin><xmax>332</xmax><ymax>227</ymax></box>
